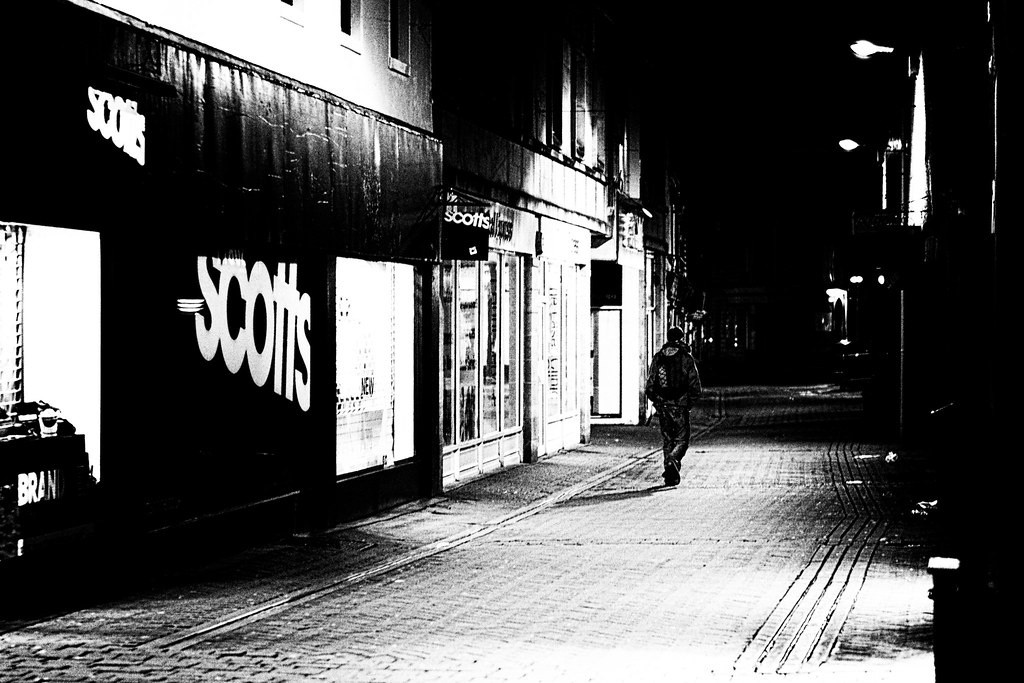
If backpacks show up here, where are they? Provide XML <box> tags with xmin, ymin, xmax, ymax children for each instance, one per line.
<box><xmin>653</xmin><ymin>350</ymin><xmax>687</xmax><ymax>402</ymax></box>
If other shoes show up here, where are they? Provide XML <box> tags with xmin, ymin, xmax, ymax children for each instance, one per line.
<box><xmin>662</xmin><ymin>459</ymin><xmax>681</xmax><ymax>485</ymax></box>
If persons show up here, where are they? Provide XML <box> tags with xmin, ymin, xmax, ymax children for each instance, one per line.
<box><xmin>643</xmin><ymin>326</ymin><xmax>702</xmax><ymax>487</ymax></box>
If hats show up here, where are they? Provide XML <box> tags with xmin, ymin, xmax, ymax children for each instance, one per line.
<box><xmin>664</xmin><ymin>328</ymin><xmax>685</xmax><ymax>340</ymax></box>
